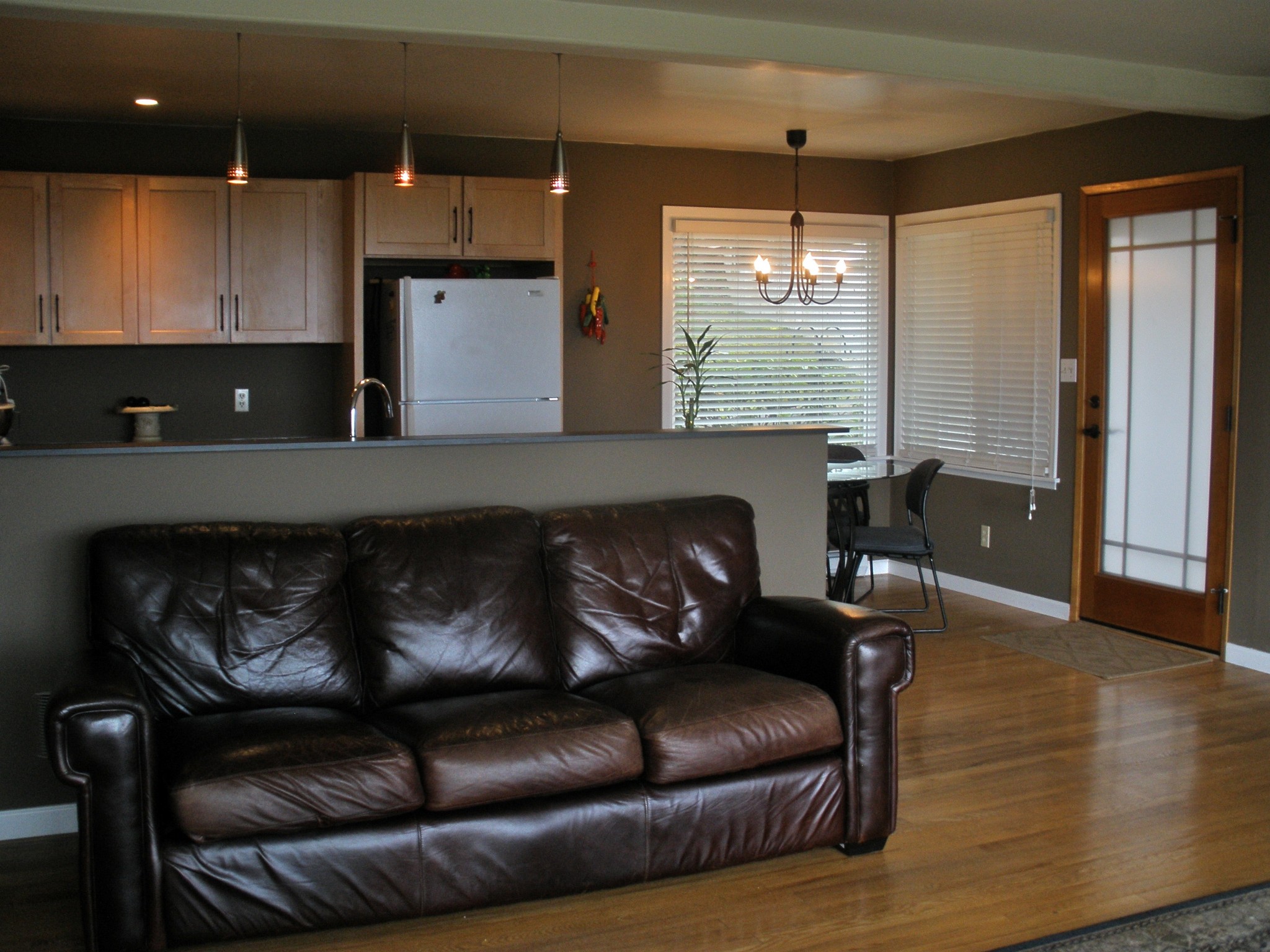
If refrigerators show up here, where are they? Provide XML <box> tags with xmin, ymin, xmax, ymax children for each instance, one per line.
<box><xmin>369</xmin><ymin>277</ymin><xmax>565</xmax><ymax>437</ymax></box>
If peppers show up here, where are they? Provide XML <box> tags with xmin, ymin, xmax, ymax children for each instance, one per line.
<box><xmin>579</xmin><ymin>287</ymin><xmax>610</xmax><ymax>345</ymax></box>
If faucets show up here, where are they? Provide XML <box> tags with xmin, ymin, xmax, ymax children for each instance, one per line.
<box><xmin>349</xmin><ymin>378</ymin><xmax>394</xmax><ymax>438</ymax></box>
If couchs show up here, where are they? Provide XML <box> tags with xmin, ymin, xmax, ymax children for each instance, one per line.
<box><xmin>47</xmin><ymin>492</ymin><xmax>916</xmax><ymax>951</ymax></box>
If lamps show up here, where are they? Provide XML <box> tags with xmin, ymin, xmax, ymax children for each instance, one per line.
<box><xmin>393</xmin><ymin>42</ymin><xmax>415</xmax><ymax>190</ymax></box>
<box><xmin>550</xmin><ymin>55</ymin><xmax>572</xmax><ymax>197</ymax></box>
<box><xmin>754</xmin><ymin>130</ymin><xmax>846</xmax><ymax>305</ymax></box>
<box><xmin>226</xmin><ymin>32</ymin><xmax>249</xmax><ymax>185</ymax></box>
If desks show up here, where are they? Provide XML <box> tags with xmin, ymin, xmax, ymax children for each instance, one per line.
<box><xmin>826</xmin><ymin>456</ymin><xmax>917</xmax><ymax>603</ymax></box>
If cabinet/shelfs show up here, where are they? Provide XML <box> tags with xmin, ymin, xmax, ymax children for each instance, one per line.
<box><xmin>365</xmin><ymin>174</ymin><xmax>554</xmax><ymax>257</ymax></box>
<box><xmin>1</xmin><ymin>180</ymin><xmax>344</xmax><ymax>345</ymax></box>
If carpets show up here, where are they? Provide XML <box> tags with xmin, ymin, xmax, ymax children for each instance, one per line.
<box><xmin>979</xmin><ymin>620</ymin><xmax>1219</xmax><ymax>680</ymax></box>
<box><xmin>990</xmin><ymin>883</ymin><xmax>1270</xmax><ymax>952</ymax></box>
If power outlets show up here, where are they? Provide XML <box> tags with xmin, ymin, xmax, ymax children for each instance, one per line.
<box><xmin>980</xmin><ymin>525</ymin><xmax>990</xmax><ymax>549</ymax></box>
<box><xmin>234</xmin><ymin>388</ymin><xmax>248</xmax><ymax>413</ymax></box>
<box><xmin>1060</xmin><ymin>358</ymin><xmax>1077</xmax><ymax>383</ymax></box>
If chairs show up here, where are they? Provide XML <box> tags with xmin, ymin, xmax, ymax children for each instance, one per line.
<box><xmin>827</xmin><ymin>444</ymin><xmax>947</xmax><ymax>632</ymax></box>
